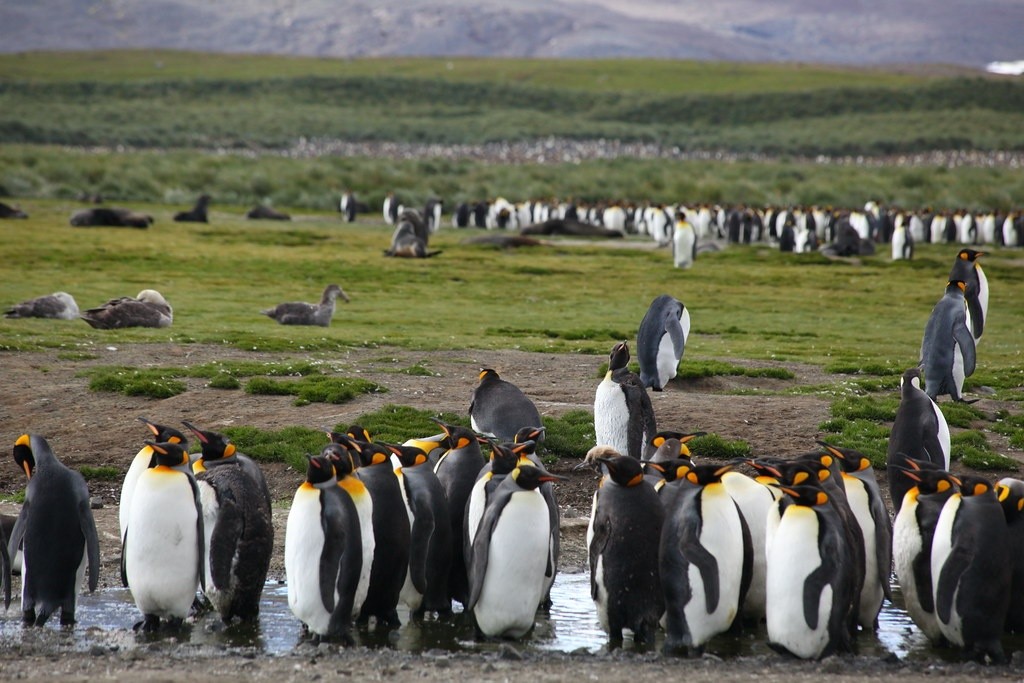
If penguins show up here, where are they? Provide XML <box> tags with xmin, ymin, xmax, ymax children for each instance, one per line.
<box><xmin>917</xmin><ymin>280</ymin><xmax>982</xmax><ymax>405</ymax></box>
<box><xmin>0</xmin><ymin>186</ymin><xmax>1022</xmax><ymax>271</ymax></box>
<box><xmin>636</xmin><ymin>293</ymin><xmax>691</xmax><ymax>392</ymax></box>
<box><xmin>0</xmin><ymin>340</ymin><xmax>1022</xmax><ymax>668</ymax></box>
<box><xmin>945</xmin><ymin>248</ymin><xmax>989</xmax><ymax>345</ymax></box>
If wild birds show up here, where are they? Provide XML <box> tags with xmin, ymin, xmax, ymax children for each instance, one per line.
<box><xmin>261</xmin><ymin>285</ymin><xmax>351</xmax><ymax>328</ymax></box>
<box><xmin>1</xmin><ymin>291</ymin><xmax>78</xmax><ymax>320</ymax></box>
<box><xmin>79</xmin><ymin>288</ymin><xmax>174</xmax><ymax>329</ymax></box>
